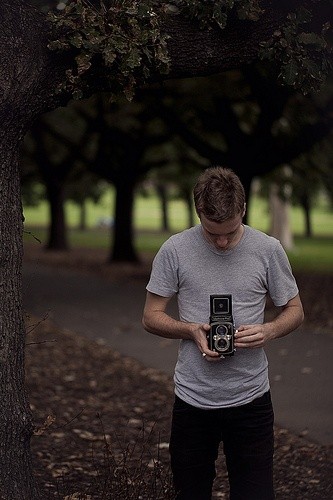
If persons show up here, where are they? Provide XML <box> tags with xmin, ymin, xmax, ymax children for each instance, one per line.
<box><xmin>142</xmin><ymin>167</ymin><xmax>304</xmax><ymax>500</ymax></box>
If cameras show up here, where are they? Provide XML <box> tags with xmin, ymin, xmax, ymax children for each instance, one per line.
<box><xmin>202</xmin><ymin>294</ymin><xmax>238</xmax><ymax>359</ymax></box>
